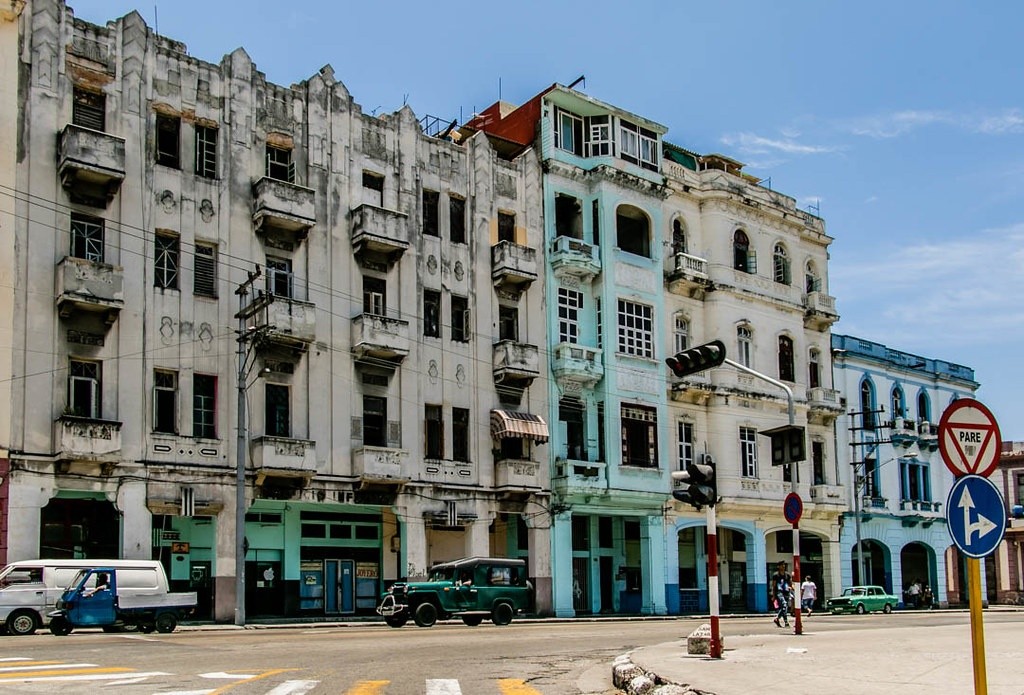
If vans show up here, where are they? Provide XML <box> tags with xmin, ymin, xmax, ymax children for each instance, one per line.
<box><xmin>0</xmin><ymin>556</ymin><xmax>170</xmax><ymax>636</ymax></box>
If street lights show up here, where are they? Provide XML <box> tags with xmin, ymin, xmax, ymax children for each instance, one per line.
<box><xmin>852</xmin><ymin>452</ymin><xmax>918</xmax><ymax>586</ymax></box>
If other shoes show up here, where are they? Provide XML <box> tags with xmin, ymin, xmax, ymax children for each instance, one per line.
<box><xmin>785</xmin><ymin>623</ymin><xmax>789</xmax><ymax>627</ymax></box>
<box><xmin>774</xmin><ymin>619</ymin><xmax>782</xmax><ymax>628</ymax></box>
<box><xmin>807</xmin><ymin>609</ymin><xmax>812</xmax><ymax>616</ymax></box>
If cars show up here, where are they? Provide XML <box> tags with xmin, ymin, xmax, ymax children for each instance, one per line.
<box><xmin>824</xmin><ymin>584</ymin><xmax>899</xmax><ymax>616</ymax></box>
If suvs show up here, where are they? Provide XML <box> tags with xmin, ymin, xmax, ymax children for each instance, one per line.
<box><xmin>375</xmin><ymin>555</ymin><xmax>536</xmax><ymax>629</ymax></box>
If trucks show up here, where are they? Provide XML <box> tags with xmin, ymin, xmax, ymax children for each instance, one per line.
<box><xmin>46</xmin><ymin>567</ymin><xmax>199</xmax><ymax>637</ymax></box>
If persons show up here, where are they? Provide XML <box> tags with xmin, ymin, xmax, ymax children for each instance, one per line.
<box><xmin>771</xmin><ymin>560</ymin><xmax>817</xmax><ymax>627</ymax></box>
<box><xmin>461</xmin><ymin>570</ymin><xmax>503</xmax><ymax>586</ymax></box>
<box><xmin>85</xmin><ymin>574</ymin><xmax>110</xmax><ymax>599</ymax></box>
<box><xmin>907</xmin><ymin>579</ymin><xmax>933</xmax><ymax>611</ymax></box>
<box><xmin>28</xmin><ymin>569</ymin><xmax>41</xmax><ymax>584</ymax></box>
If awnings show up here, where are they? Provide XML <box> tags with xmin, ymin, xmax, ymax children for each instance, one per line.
<box><xmin>489</xmin><ymin>408</ymin><xmax>550</xmax><ymax>445</ymax></box>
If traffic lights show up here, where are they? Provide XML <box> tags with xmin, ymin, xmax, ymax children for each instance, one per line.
<box><xmin>671</xmin><ymin>462</ymin><xmax>717</xmax><ymax>510</ymax></box>
<box><xmin>664</xmin><ymin>339</ymin><xmax>726</xmax><ymax>377</ymax></box>
